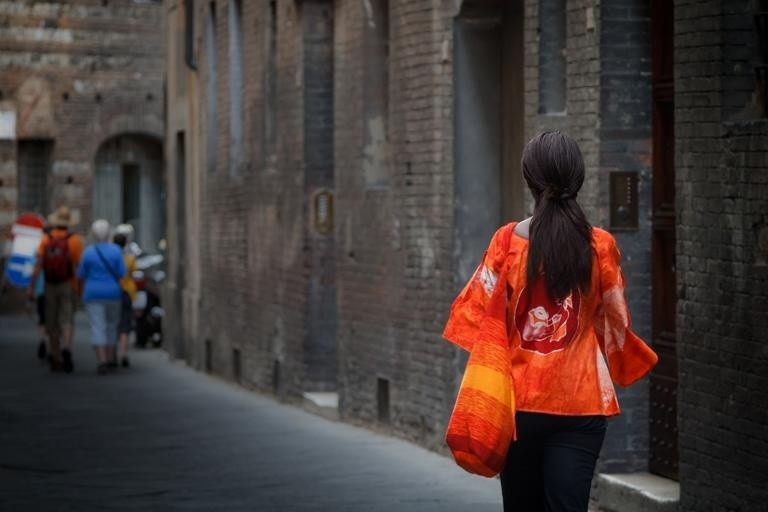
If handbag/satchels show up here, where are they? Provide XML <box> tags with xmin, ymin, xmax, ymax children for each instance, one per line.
<box><xmin>444</xmin><ymin>221</ymin><xmax>518</xmax><ymax>479</ymax></box>
<box><xmin>122</xmin><ymin>291</ymin><xmax>132</xmax><ymax>312</ymax></box>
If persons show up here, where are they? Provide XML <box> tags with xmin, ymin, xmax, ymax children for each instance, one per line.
<box><xmin>441</xmin><ymin>132</ymin><xmax>659</xmax><ymax>512</ymax></box>
<box><xmin>20</xmin><ymin>203</ymin><xmax>164</xmax><ymax>374</ymax></box>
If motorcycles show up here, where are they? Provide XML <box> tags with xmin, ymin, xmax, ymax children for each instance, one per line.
<box><xmin>128</xmin><ymin>238</ymin><xmax>167</xmax><ymax>348</ymax></box>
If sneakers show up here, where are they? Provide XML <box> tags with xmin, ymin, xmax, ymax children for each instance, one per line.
<box><xmin>37</xmin><ymin>341</ymin><xmax>132</xmax><ymax>376</ymax></box>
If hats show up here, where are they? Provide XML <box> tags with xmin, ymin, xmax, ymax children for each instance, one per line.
<box><xmin>47</xmin><ymin>206</ymin><xmax>81</xmax><ymax>227</ymax></box>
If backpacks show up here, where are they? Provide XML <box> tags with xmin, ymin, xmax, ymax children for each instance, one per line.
<box><xmin>42</xmin><ymin>231</ymin><xmax>74</xmax><ymax>285</ymax></box>
